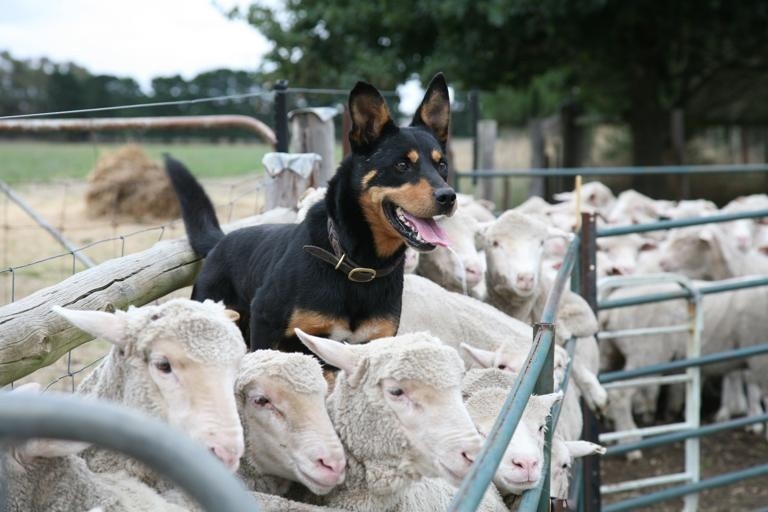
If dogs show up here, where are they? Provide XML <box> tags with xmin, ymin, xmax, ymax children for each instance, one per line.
<box><xmin>158</xmin><ymin>64</ymin><xmax>460</xmax><ymax>350</ymax></box>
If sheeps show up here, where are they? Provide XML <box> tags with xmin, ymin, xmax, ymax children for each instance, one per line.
<box><xmin>0</xmin><ymin>181</ymin><xmax>768</xmax><ymax>512</ymax></box>
